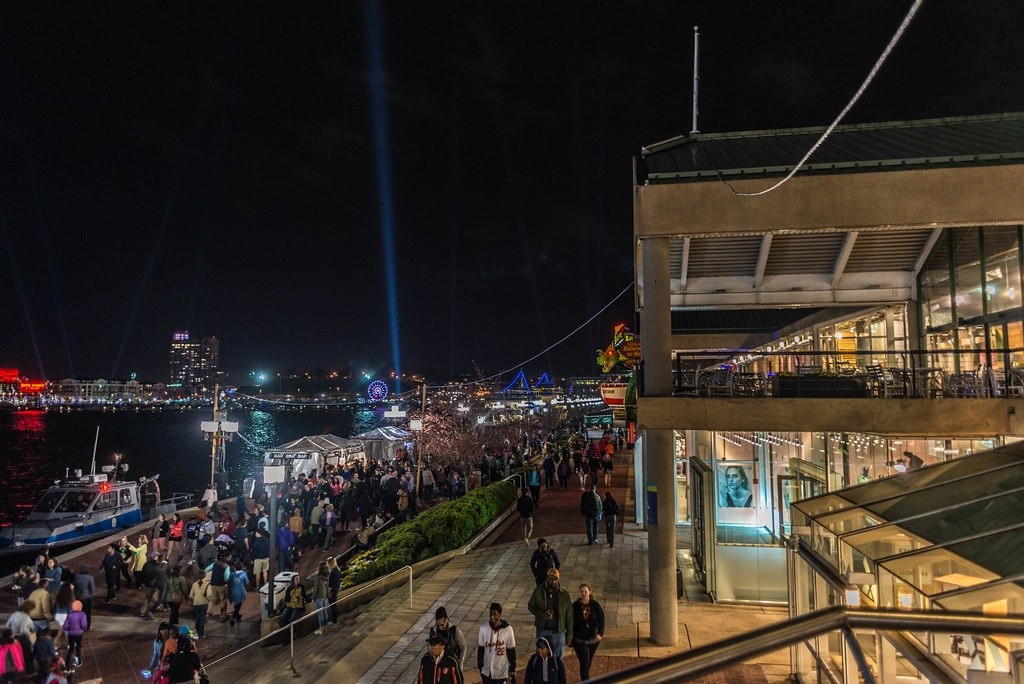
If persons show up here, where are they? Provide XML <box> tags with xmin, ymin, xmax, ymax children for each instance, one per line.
<box><xmin>476</xmin><ymin>603</ymin><xmax>516</xmax><ymax>684</ymax></box>
<box><xmin>418</xmin><ymin>633</ymin><xmax>465</xmax><ymax>684</ymax></box>
<box><xmin>523</xmin><ymin>636</ymin><xmax>567</xmax><ymax>684</ymax></box>
<box><xmin>879</xmin><ymin>474</ymin><xmax>884</xmax><ymax>479</ymax></box>
<box><xmin>564</xmin><ymin>583</ymin><xmax>605</xmax><ymax>682</ymax></box>
<box><xmin>723</xmin><ymin>360</ymin><xmax>740</xmax><ymax>394</ymax></box>
<box><xmin>857</xmin><ymin>467</ymin><xmax>873</xmax><ymax>484</ymax></box>
<box><xmin>530</xmin><ymin>538</ymin><xmax>562</xmax><ymax>588</ymax></box>
<box><xmin>0</xmin><ymin>464</ymin><xmax>344</xmax><ymax>684</ymax></box>
<box><xmin>334</xmin><ymin>421</ymin><xmax>628</xmax><ymax>548</ymax></box>
<box><xmin>720</xmin><ymin>466</ymin><xmax>753</xmax><ymax>507</ymax></box>
<box><xmin>528</xmin><ymin>568</ymin><xmax>573</xmax><ymax>665</ymax></box>
<box><xmin>429</xmin><ymin>606</ymin><xmax>467</xmax><ymax>672</ymax></box>
<box><xmin>903</xmin><ymin>451</ymin><xmax>924</xmax><ymax>472</ymax></box>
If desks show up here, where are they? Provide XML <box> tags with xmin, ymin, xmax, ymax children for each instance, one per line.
<box><xmin>888</xmin><ymin>368</ymin><xmax>942</xmax><ymax>399</ymax></box>
<box><xmin>734</xmin><ymin>372</ymin><xmax>756</xmax><ymax>396</ymax></box>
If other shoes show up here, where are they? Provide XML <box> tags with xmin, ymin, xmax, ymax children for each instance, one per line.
<box><xmin>163</xmin><ymin>606</ymin><xmax>171</xmax><ymax>611</ymax></box>
<box><xmin>219</xmin><ymin>614</ymin><xmax>230</xmax><ymax>622</ymax></box>
<box><xmin>147</xmin><ymin>611</ymin><xmax>154</xmax><ymax>619</ymax></box>
<box><xmin>594</xmin><ymin>539</ymin><xmax>599</xmax><ymax>543</ymax></box>
<box><xmin>192</xmin><ymin>556</ymin><xmax>196</xmax><ymax>562</ymax></box>
<box><xmin>161</xmin><ymin>557</ymin><xmax>169</xmax><ymax>563</ymax></box>
<box><xmin>104</xmin><ymin>595</ymin><xmax>117</xmax><ymax>604</ymax></box>
<box><xmin>76</xmin><ymin>660</ymin><xmax>83</xmax><ymax>667</ymax></box>
<box><xmin>523</xmin><ymin>537</ymin><xmax>530</xmax><ymax>546</ymax></box>
<box><xmin>24</xmin><ymin>671</ymin><xmax>38</xmax><ymax>677</ymax></box>
<box><xmin>206</xmin><ymin>613</ymin><xmax>210</xmax><ymax>620</ymax></box>
<box><xmin>254</xmin><ymin>586</ymin><xmax>261</xmax><ymax>591</ymax></box>
<box><xmin>322</xmin><ymin>549</ymin><xmax>328</xmax><ymax>552</ymax></box>
<box><xmin>187</xmin><ymin>559</ymin><xmax>193</xmax><ymax>565</ymax></box>
<box><xmin>178</xmin><ymin>555</ymin><xmax>183</xmax><ymax>560</ymax></box>
<box><xmin>64</xmin><ymin>669</ymin><xmax>72</xmax><ymax>674</ymax></box>
<box><xmin>313</xmin><ymin>627</ymin><xmax>325</xmax><ymax>634</ymax></box>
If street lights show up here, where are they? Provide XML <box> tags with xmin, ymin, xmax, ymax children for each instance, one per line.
<box><xmin>200</xmin><ymin>421</ymin><xmax>238</xmax><ymax>490</ymax></box>
<box><xmin>264</xmin><ymin>465</ymin><xmax>287</xmax><ymax>617</ymax></box>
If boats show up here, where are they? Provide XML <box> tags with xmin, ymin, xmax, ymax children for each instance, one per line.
<box><xmin>0</xmin><ymin>426</ymin><xmax>194</xmax><ymax>551</ymax></box>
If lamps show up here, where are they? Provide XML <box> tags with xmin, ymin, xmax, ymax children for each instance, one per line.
<box><xmin>845</xmin><ymin>572</ymin><xmax>875</xmax><ymax>606</ymax></box>
<box><xmin>789</xmin><ymin>526</ymin><xmax>811</xmax><ymax>552</ymax></box>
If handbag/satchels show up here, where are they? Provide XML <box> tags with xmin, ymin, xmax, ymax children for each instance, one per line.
<box><xmin>171</xmin><ymin>591</ymin><xmax>182</xmax><ymax>605</ymax></box>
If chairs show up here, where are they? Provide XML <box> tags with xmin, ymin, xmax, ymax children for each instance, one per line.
<box><xmin>682</xmin><ymin>364</ymin><xmax>772</xmax><ymax>397</ymax></box>
<box><xmin>796</xmin><ymin>362</ymin><xmax>1024</xmax><ymax>399</ymax></box>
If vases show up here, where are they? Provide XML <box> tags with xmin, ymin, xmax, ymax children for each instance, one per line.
<box><xmin>772</xmin><ymin>378</ymin><xmax>867</xmax><ymax>398</ymax></box>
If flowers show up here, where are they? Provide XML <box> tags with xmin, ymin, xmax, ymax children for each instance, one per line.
<box><xmin>767</xmin><ymin>371</ymin><xmax>839</xmax><ymax>378</ymax></box>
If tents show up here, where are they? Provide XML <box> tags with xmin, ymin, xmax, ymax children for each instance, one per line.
<box><xmin>261</xmin><ymin>425</ymin><xmax>414</xmax><ymax>497</ymax></box>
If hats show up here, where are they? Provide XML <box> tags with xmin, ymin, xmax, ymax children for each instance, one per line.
<box><xmin>179</xmin><ymin>624</ymin><xmax>190</xmax><ymax>635</ymax></box>
<box><xmin>425</xmin><ymin>632</ymin><xmax>446</xmax><ymax>645</ymax></box>
<box><xmin>71</xmin><ymin>600</ymin><xmax>83</xmax><ymax>611</ymax></box>
<box><xmin>148</xmin><ymin>551</ymin><xmax>160</xmax><ymax>557</ymax></box>
<box><xmin>547</xmin><ymin>568</ymin><xmax>560</xmax><ymax>578</ymax></box>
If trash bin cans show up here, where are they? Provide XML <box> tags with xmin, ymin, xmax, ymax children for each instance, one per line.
<box><xmin>260</xmin><ymin>570</ymin><xmax>299</xmax><ymax>620</ymax></box>
